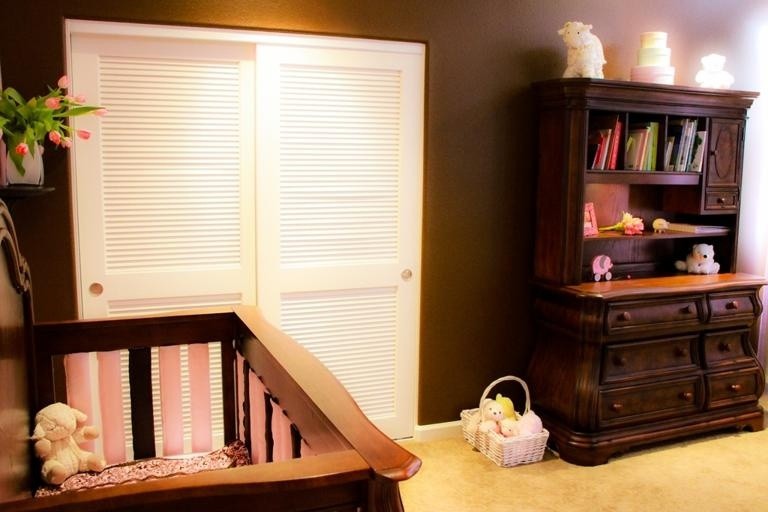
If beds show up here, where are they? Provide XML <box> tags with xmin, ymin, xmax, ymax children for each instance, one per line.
<box><xmin>0</xmin><ymin>197</ymin><xmax>422</xmax><ymax>511</ymax></box>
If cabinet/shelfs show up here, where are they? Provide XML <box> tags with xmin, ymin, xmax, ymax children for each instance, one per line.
<box><xmin>520</xmin><ymin>77</ymin><xmax>768</xmax><ymax>466</ymax></box>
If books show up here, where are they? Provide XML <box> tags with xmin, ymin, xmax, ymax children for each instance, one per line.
<box><xmin>667</xmin><ymin>223</ymin><xmax>731</xmax><ymax>235</ymax></box>
<box><xmin>586</xmin><ymin>114</ymin><xmax>708</xmax><ymax>174</ymax></box>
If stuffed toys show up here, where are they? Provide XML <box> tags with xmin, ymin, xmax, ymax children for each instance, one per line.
<box><xmin>28</xmin><ymin>401</ymin><xmax>106</xmax><ymax>485</ymax></box>
<box><xmin>466</xmin><ymin>392</ymin><xmax>543</xmax><ymax>441</ymax></box>
<box><xmin>673</xmin><ymin>242</ymin><xmax>721</xmax><ymax>276</ymax></box>
<box><xmin>558</xmin><ymin>20</ymin><xmax>609</xmax><ymax>80</ymax></box>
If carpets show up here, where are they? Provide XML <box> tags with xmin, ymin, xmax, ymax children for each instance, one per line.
<box><xmin>380</xmin><ymin>423</ymin><xmax>768</xmax><ymax>512</ymax></box>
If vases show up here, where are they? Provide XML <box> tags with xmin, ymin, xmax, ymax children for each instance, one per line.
<box><xmin>4</xmin><ymin>130</ymin><xmax>44</xmax><ymax>187</ymax></box>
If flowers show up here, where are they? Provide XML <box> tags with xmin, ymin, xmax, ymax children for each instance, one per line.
<box><xmin>0</xmin><ymin>78</ymin><xmax>109</xmax><ymax>175</ymax></box>
